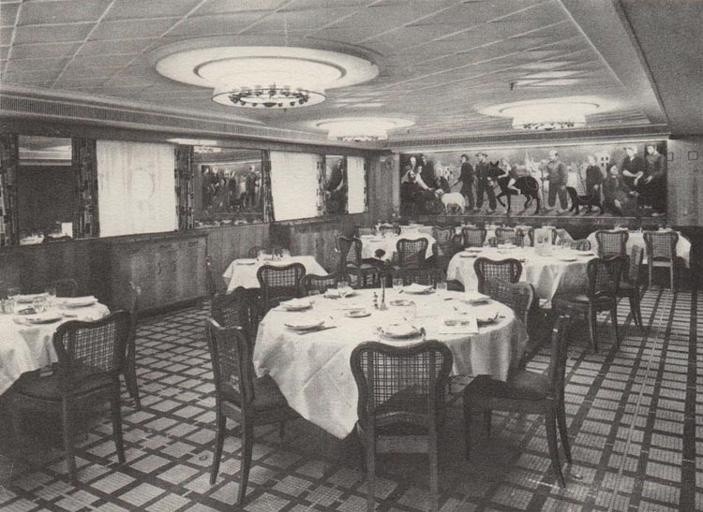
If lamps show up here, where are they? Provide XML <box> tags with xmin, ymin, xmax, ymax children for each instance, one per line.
<box><xmin>471</xmin><ymin>94</ymin><xmax>615</xmax><ymax>135</ymax></box>
<box><xmin>143</xmin><ymin>32</ymin><xmax>415</xmax><ymax>146</ymax></box>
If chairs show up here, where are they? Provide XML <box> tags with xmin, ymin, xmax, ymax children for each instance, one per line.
<box><xmin>0</xmin><ymin>277</ymin><xmax>141</xmax><ymax>489</ymax></box>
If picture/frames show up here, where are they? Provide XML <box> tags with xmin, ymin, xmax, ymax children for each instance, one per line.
<box><xmin>322</xmin><ymin>151</ymin><xmax>348</xmax><ymax>216</ymax></box>
<box><xmin>193</xmin><ymin>152</ymin><xmax>263</xmax><ymax>221</ymax></box>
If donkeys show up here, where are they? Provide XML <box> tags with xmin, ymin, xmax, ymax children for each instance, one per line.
<box><xmin>487</xmin><ymin>160</ymin><xmax>541</xmax><ymax>215</ymax></box>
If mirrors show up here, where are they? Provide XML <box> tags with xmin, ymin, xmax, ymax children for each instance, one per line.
<box><xmin>2</xmin><ymin>132</ymin><xmax>85</xmax><ymax>245</ymax></box>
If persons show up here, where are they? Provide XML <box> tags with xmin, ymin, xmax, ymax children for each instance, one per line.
<box><xmin>397</xmin><ymin>142</ymin><xmax>667</xmax><ymax>218</ymax></box>
<box><xmin>324</xmin><ymin>157</ymin><xmax>344</xmax><ymax>214</ymax></box>
<box><xmin>200</xmin><ymin>162</ymin><xmax>261</xmax><ymax>215</ymax></box>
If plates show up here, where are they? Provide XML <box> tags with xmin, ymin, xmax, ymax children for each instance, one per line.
<box><xmin>0</xmin><ymin>285</ymin><xmax>99</xmax><ymax>325</ymax></box>
<box><xmin>277</xmin><ymin>274</ymin><xmax>500</xmax><ymax>339</ymax></box>
<box><xmin>360</xmin><ymin>232</ymin><xmax>383</xmax><ymax>243</ymax></box>
<box><xmin>458</xmin><ymin>232</ymin><xmax>595</xmax><ymax>263</ymax></box>
<box><xmin>235</xmin><ymin>247</ymin><xmax>292</xmax><ymax>266</ymax></box>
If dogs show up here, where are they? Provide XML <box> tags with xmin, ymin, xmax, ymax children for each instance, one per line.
<box><xmin>434</xmin><ymin>188</ymin><xmax>466</xmax><ymax>216</ymax></box>
<box><xmin>566</xmin><ymin>186</ymin><xmax>605</xmax><ymax>216</ymax></box>
